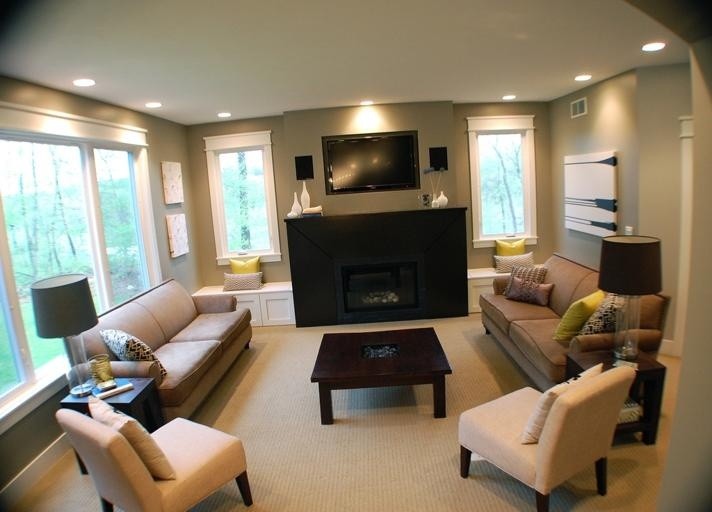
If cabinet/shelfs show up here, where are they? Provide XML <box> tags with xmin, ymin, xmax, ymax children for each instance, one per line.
<box><xmin>467</xmin><ymin>263</ymin><xmax>544</xmax><ymax>313</ymax></box>
<box><xmin>192</xmin><ymin>281</ymin><xmax>296</xmax><ymax>326</ymax></box>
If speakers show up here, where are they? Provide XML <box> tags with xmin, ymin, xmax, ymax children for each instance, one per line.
<box><xmin>428</xmin><ymin>146</ymin><xmax>449</xmax><ymax>172</ymax></box>
<box><xmin>292</xmin><ymin>155</ymin><xmax>316</xmax><ymax>180</ymax></box>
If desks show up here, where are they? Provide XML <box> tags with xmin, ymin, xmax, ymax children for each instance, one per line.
<box><xmin>562</xmin><ymin>348</ymin><xmax>666</xmax><ymax>445</ymax></box>
<box><xmin>60</xmin><ymin>377</ymin><xmax>164</xmax><ymax>475</ymax></box>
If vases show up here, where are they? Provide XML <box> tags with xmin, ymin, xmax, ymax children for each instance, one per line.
<box><xmin>431</xmin><ymin>191</ymin><xmax>448</xmax><ymax>208</ymax></box>
<box><xmin>291</xmin><ymin>180</ymin><xmax>311</xmax><ymax>216</ymax></box>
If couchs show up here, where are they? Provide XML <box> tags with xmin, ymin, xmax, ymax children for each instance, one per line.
<box><xmin>479</xmin><ymin>253</ymin><xmax>671</xmax><ymax>393</ymax></box>
<box><xmin>63</xmin><ymin>278</ymin><xmax>252</xmax><ymax>423</ymax></box>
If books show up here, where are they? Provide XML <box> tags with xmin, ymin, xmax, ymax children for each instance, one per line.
<box><xmin>91</xmin><ymin>378</ymin><xmax>133</xmax><ymax>399</ymax></box>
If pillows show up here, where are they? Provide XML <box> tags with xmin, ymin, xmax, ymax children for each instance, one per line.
<box><xmin>521</xmin><ymin>362</ymin><xmax>603</xmax><ymax>444</ymax></box>
<box><xmin>493</xmin><ymin>240</ymin><xmax>624</xmax><ymax>341</ymax></box>
<box><xmin>87</xmin><ymin>395</ymin><xmax>176</xmax><ymax>481</ymax></box>
<box><xmin>223</xmin><ymin>256</ymin><xmax>263</xmax><ymax>291</ymax></box>
<box><xmin>99</xmin><ymin>329</ymin><xmax>167</xmax><ymax>378</ymax></box>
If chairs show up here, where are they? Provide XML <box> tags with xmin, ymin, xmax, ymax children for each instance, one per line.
<box><xmin>458</xmin><ymin>366</ymin><xmax>636</xmax><ymax>512</ymax></box>
<box><xmin>55</xmin><ymin>408</ymin><xmax>253</xmax><ymax>512</ymax></box>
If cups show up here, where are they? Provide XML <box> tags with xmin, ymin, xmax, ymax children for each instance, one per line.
<box><xmin>88</xmin><ymin>354</ymin><xmax>114</xmax><ymax>385</ymax></box>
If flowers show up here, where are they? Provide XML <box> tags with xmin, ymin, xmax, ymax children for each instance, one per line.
<box><xmin>422</xmin><ymin>167</ymin><xmax>445</xmax><ymax>193</ymax></box>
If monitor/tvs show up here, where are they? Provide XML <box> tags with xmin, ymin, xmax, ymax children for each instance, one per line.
<box><xmin>321</xmin><ymin>129</ymin><xmax>421</xmax><ymax>196</ymax></box>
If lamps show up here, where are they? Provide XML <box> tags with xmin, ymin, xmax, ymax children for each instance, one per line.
<box><xmin>598</xmin><ymin>235</ymin><xmax>663</xmax><ymax>361</ymax></box>
<box><xmin>31</xmin><ymin>274</ymin><xmax>98</xmax><ymax>398</ymax></box>
